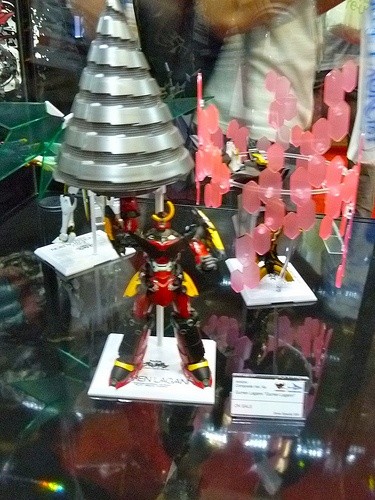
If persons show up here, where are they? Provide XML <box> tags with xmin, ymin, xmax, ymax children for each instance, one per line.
<box><xmin>190</xmin><ymin>0</ymin><xmax>346</xmax><ymax>207</ymax></box>
<box><xmin>73</xmin><ymin>0</ymin><xmax>221</xmax><ymax>207</ymax></box>
